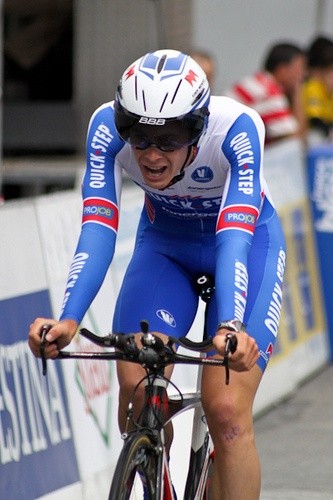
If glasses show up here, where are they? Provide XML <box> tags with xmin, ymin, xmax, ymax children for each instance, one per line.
<box><xmin>133</xmin><ymin>141</ymin><xmax>181</xmax><ymax>152</ymax></box>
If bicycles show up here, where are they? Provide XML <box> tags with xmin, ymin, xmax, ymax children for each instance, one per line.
<box><xmin>38</xmin><ymin>320</ymin><xmax>247</xmax><ymax>499</ymax></box>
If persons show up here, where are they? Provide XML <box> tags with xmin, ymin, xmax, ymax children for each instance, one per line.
<box><xmin>27</xmin><ymin>48</ymin><xmax>287</xmax><ymax>500</ymax></box>
<box><xmin>180</xmin><ymin>32</ymin><xmax>333</xmax><ymax>151</ymax></box>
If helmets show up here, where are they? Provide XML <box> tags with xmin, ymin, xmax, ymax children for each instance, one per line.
<box><xmin>113</xmin><ymin>49</ymin><xmax>211</xmax><ymax>152</ymax></box>
